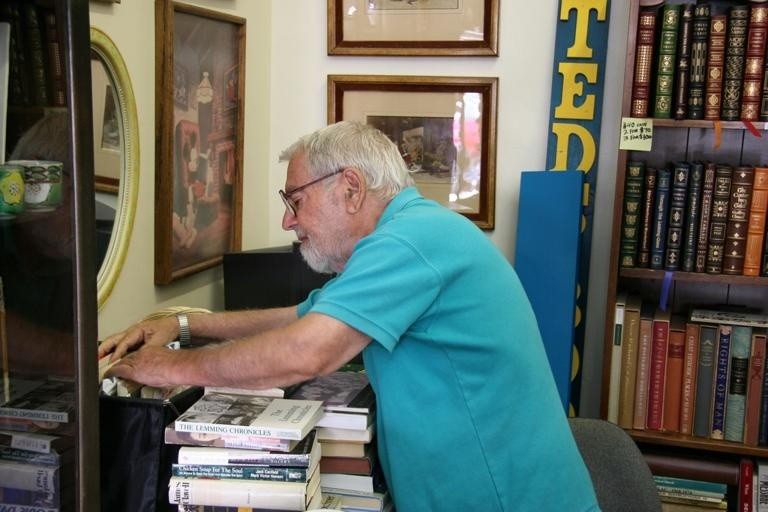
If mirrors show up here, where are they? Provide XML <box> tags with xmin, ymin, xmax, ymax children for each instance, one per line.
<box><xmin>89</xmin><ymin>27</ymin><xmax>141</xmax><ymax>308</ymax></box>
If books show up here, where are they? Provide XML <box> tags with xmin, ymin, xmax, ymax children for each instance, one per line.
<box><xmin>619</xmin><ymin>158</ymin><xmax>768</xmax><ymax>277</ymax></box>
<box><xmin>164</xmin><ymin>365</ymin><xmax>395</xmax><ymax>512</ymax></box>
<box><xmin>638</xmin><ymin>451</ymin><xmax>768</xmax><ymax>512</ymax></box>
<box><xmin>2</xmin><ymin>379</ymin><xmax>80</xmax><ymax>511</ymax></box>
<box><xmin>606</xmin><ymin>295</ymin><xmax>768</xmax><ymax>447</ymax></box>
<box><xmin>631</xmin><ymin>1</ymin><xmax>768</xmax><ymax>122</ymax></box>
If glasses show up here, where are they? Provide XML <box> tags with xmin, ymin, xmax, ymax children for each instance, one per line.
<box><xmin>279</xmin><ymin>168</ymin><xmax>346</xmax><ymax>218</ymax></box>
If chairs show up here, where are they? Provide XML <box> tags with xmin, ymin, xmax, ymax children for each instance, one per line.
<box><xmin>570</xmin><ymin>419</ymin><xmax>667</xmax><ymax>512</ymax></box>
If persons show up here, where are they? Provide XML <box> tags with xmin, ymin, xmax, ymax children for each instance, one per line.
<box><xmin>98</xmin><ymin>118</ymin><xmax>604</xmax><ymax>512</ymax></box>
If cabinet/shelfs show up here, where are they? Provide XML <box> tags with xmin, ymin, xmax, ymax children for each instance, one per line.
<box><xmin>598</xmin><ymin>0</ymin><xmax>768</xmax><ymax>512</ymax></box>
<box><xmin>0</xmin><ymin>0</ymin><xmax>99</xmax><ymax>512</ymax></box>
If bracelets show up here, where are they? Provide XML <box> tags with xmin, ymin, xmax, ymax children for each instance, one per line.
<box><xmin>177</xmin><ymin>313</ymin><xmax>191</xmax><ymax>347</ymax></box>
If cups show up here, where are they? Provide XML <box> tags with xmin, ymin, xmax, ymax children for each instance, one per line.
<box><xmin>0</xmin><ymin>160</ymin><xmax>64</xmax><ymax>220</ymax></box>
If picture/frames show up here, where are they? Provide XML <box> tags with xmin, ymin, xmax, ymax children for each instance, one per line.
<box><xmin>327</xmin><ymin>0</ymin><xmax>500</xmax><ymax>57</ymax></box>
<box><xmin>155</xmin><ymin>0</ymin><xmax>247</xmax><ymax>285</ymax></box>
<box><xmin>326</xmin><ymin>75</ymin><xmax>498</xmax><ymax>229</ymax></box>
